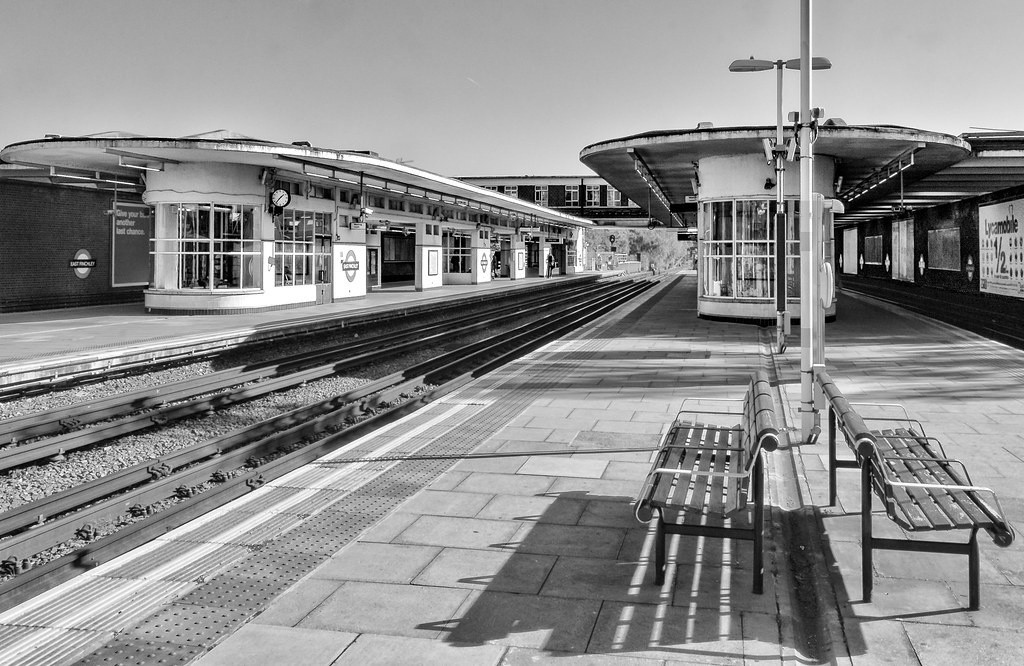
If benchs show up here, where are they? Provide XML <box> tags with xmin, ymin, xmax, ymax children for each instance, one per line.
<box><xmin>634</xmin><ymin>370</ymin><xmax>781</xmax><ymax>595</ymax></box>
<box><xmin>815</xmin><ymin>372</ymin><xmax>1015</xmax><ymax>609</ymax></box>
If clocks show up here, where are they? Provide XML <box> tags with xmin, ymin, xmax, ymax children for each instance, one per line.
<box><xmin>271</xmin><ymin>188</ymin><xmax>291</xmax><ymax>207</ymax></box>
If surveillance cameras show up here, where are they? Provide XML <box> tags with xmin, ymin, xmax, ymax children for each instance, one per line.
<box><xmin>363</xmin><ymin>208</ymin><xmax>373</xmax><ymax>215</ymax></box>
<box><xmin>527</xmin><ymin>233</ymin><xmax>531</xmax><ymax>237</ymax></box>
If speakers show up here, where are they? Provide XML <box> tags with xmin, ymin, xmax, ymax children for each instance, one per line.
<box><xmin>786</xmin><ymin>137</ymin><xmax>799</xmax><ymax>162</ymax></box>
<box><xmin>762</xmin><ymin>138</ymin><xmax>773</xmax><ymax>162</ymax></box>
